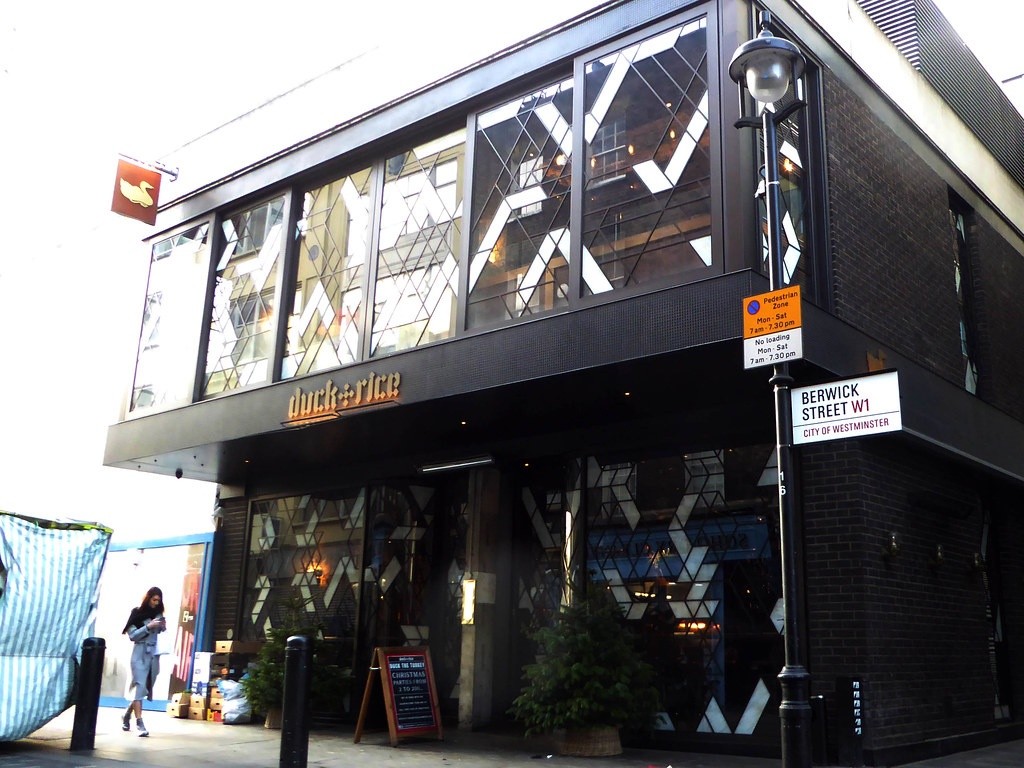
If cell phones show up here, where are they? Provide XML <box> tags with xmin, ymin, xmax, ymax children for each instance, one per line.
<box><xmin>159</xmin><ymin>617</ymin><xmax>165</xmax><ymax>621</ymax></box>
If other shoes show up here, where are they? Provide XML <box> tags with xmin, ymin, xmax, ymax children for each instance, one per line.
<box><xmin>122</xmin><ymin>715</ymin><xmax>130</xmax><ymax>731</ymax></box>
<box><xmin>137</xmin><ymin>726</ymin><xmax>149</xmax><ymax>737</ymax></box>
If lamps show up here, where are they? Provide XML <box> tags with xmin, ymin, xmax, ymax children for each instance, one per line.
<box><xmin>965</xmin><ymin>552</ymin><xmax>986</xmax><ymax>576</ymax></box>
<box><xmin>881</xmin><ymin>530</ymin><xmax>904</xmax><ymax>562</ymax></box>
<box><xmin>928</xmin><ymin>544</ymin><xmax>948</xmax><ymax>569</ymax></box>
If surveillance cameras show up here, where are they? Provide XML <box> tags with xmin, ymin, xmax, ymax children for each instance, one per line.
<box><xmin>176</xmin><ymin>471</ymin><xmax>182</xmax><ymax>479</ymax></box>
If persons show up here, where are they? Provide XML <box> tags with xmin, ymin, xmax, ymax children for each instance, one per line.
<box><xmin>122</xmin><ymin>587</ymin><xmax>167</xmax><ymax>737</ymax></box>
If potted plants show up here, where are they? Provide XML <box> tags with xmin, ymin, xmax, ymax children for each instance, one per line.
<box><xmin>244</xmin><ymin>589</ymin><xmax>346</xmax><ymax>729</ymax></box>
<box><xmin>506</xmin><ymin>582</ymin><xmax>661</xmax><ymax>756</ymax></box>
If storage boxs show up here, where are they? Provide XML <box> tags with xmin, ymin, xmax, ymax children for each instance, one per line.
<box><xmin>167</xmin><ymin>703</ymin><xmax>189</xmax><ymax>717</ymax></box>
<box><xmin>191</xmin><ymin>682</ymin><xmax>218</xmax><ymax>697</ymax></box>
<box><xmin>210</xmin><ymin>698</ymin><xmax>222</xmax><ymax>710</ymax></box>
<box><xmin>194</xmin><ymin>652</ymin><xmax>230</xmax><ymax>667</ymax></box>
<box><xmin>192</xmin><ymin>667</ymin><xmax>228</xmax><ymax>683</ymax></box>
<box><xmin>172</xmin><ymin>693</ymin><xmax>191</xmax><ymax>704</ymax></box>
<box><xmin>215</xmin><ymin>640</ymin><xmax>242</xmax><ymax>653</ymax></box>
<box><xmin>189</xmin><ymin>707</ymin><xmax>207</xmax><ymax>720</ymax></box>
<box><xmin>189</xmin><ymin>697</ymin><xmax>210</xmax><ymax>708</ymax></box>
<box><xmin>211</xmin><ymin>688</ymin><xmax>222</xmax><ymax>698</ymax></box>
